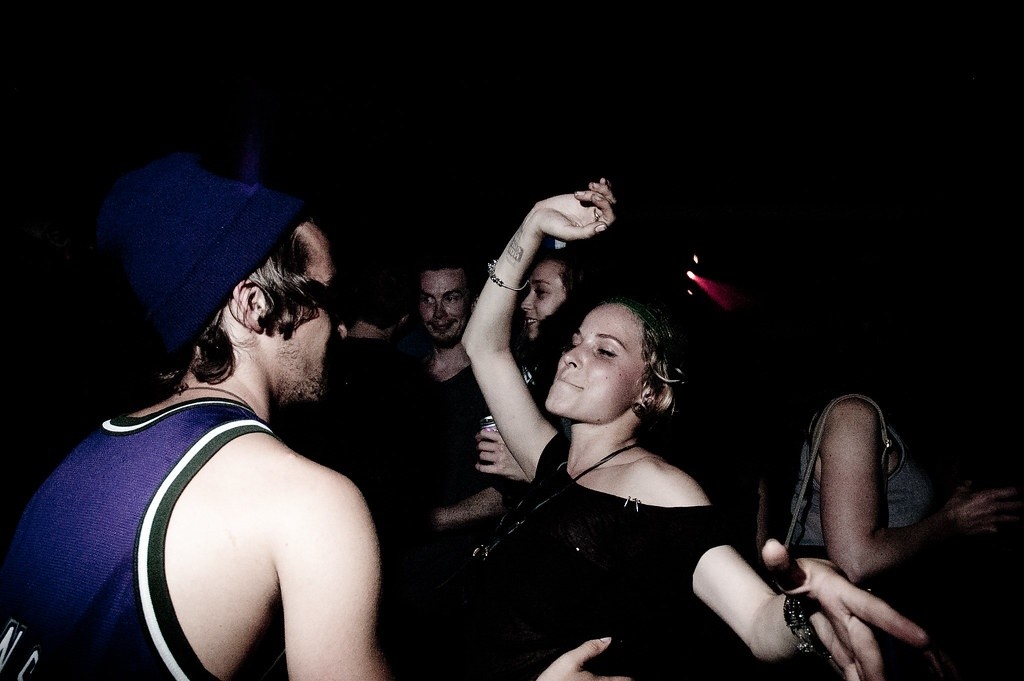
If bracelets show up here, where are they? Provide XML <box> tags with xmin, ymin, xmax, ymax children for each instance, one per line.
<box><xmin>487</xmin><ymin>259</ymin><xmax>527</xmax><ymax>290</ymax></box>
<box><xmin>783</xmin><ymin>595</ymin><xmax>833</xmax><ymax>658</ymax></box>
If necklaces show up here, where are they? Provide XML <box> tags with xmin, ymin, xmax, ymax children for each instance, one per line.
<box><xmin>473</xmin><ymin>442</ymin><xmax>637</xmax><ymax>560</ymax></box>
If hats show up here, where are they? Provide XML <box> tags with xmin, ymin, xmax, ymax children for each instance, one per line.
<box><xmin>98</xmin><ymin>151</ymin><xmax>305</xmax><ymax>355</ymax></box>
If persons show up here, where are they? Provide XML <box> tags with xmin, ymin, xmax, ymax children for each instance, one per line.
<box><xmin>459</xmin><ymin>177</ymin><xmax>928</xmax><ymax>681</ymax></box>
<box><xmin>417</xmin><ymin>253</ymin><xmax>531</xmax><ymax>530</ymax></box>
<box><xmin>786</xmin><ymin>285</ymin><xmax>1024</xmax><ymax>681</ymax></box>
<box><xmin>0</xmin><ymin>153</ymin><xmax>394</xmax><ymax>681</ymax></box>
<box><xmin>478</xmin><ymin>250</ymin><xmax>599</xmax><ymax>482</ymax></box>
<box><xmin>269</xmin><ymin>249</ymin><xmax>442</xmax><ymax>558</ymax></box>
